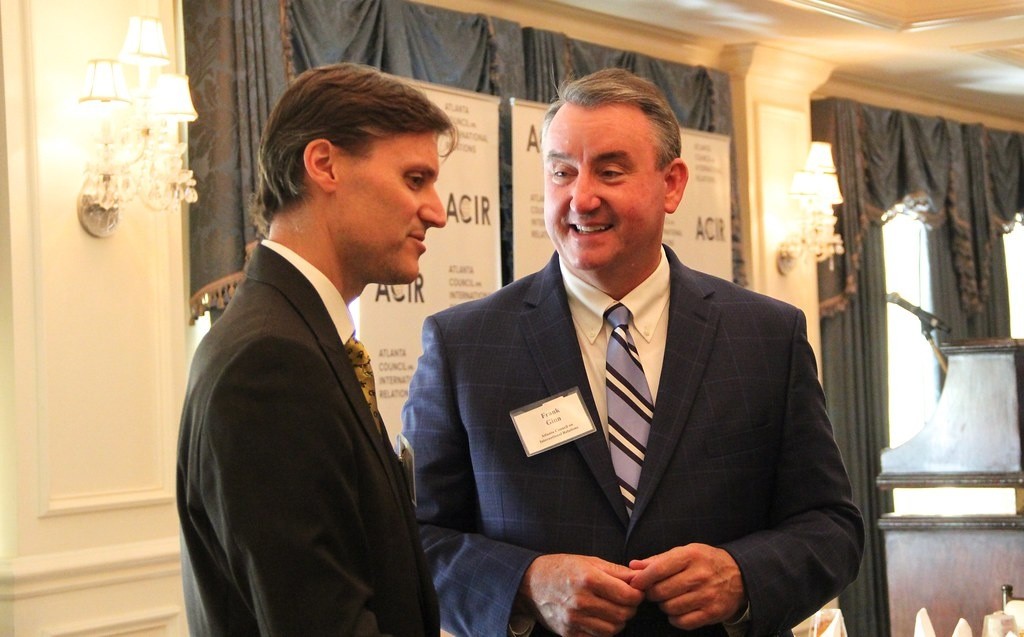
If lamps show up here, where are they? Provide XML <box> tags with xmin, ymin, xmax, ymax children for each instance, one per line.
<box><xmin>76</xmin><ymin>15</ymin><xmax>199</xmax><ymax>238</ymax></box>
<box><xmin>776</xmin><ymin>141</ymin><xmax>845</xmax><ymax>277</ymax></box>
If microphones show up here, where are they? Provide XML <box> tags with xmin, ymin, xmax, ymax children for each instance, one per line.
<box><xmin>889</xmin><ymin>293</ymin><xmax>953</xmax><ymax>334</ymax></box>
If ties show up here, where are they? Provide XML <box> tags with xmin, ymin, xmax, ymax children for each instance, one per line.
<box><xmin>344</xmin><ymin>336</ymin><xmax>381</xmax><ymax>435</ymax></box>
<box><xmin>603</xmin><ymin>303</ymin><xmax>655</xmax><ymax>519</ymax></box>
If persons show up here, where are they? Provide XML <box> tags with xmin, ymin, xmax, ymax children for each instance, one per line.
<box><xmin>174</xmin><ymin>62</ymin><xmax>461</xmax><ymax>637</ymax></box>
<box><xmin>398</xmin><ymin>67</ymin><xmax>864</xmax><ymax>637</ymax></box>
